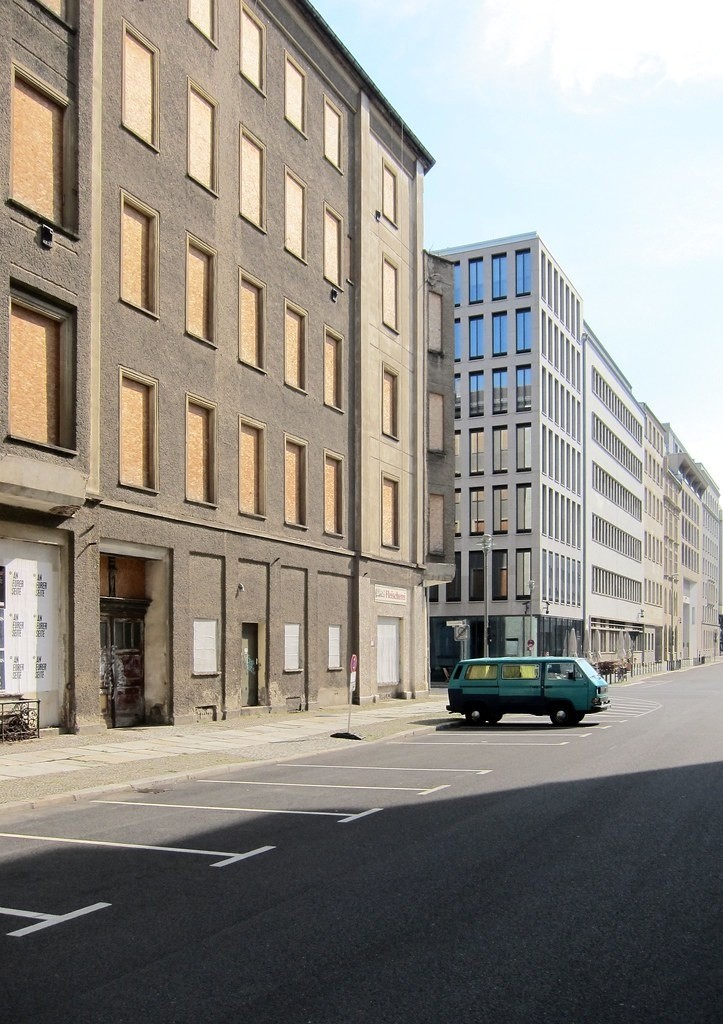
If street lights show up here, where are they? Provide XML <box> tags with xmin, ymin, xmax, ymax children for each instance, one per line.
<box><xmin>527</xmin><ymin>579</ymin><xmax>537</xmax><ymax>656</ymax></box>
<box><xmin>475</xmin><ymin>533</ymin><xmax>497</xmax><ymax>658</ymax></box>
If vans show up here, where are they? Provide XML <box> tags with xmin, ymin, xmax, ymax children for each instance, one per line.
<box><xmin>443</xmin><ymin>653</ymin><xmax>612</xmax><ymax>728</ymax></box>
